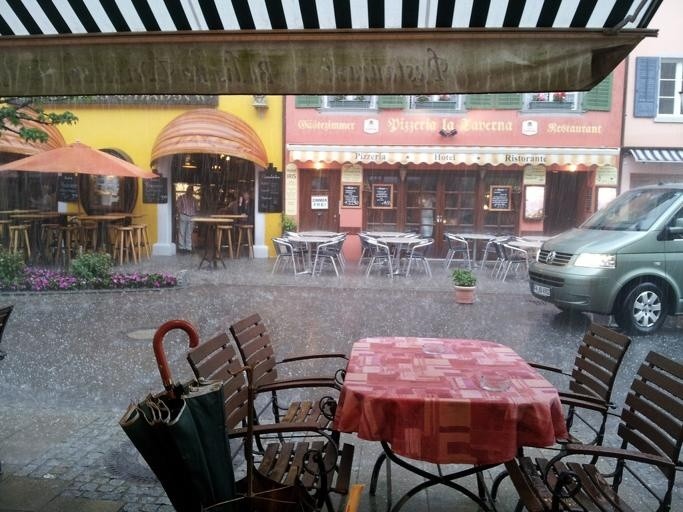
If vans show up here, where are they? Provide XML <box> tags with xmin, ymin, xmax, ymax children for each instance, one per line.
<box><xmin>527</xmin><ymin>181</ymin><xmax>683</xmax><ymax>335</ymax></box>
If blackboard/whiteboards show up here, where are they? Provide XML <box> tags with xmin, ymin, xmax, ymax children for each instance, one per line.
<box><xmin>372</xmin><ymin>184</ymin><xmax>393</xmax><ymax>210</ymax></box>
<box><xmin>489</xmin><ymin>185</ymin><xmax>512</xmax><ymax>212</ymax></box>
<box><xmin>142</xmin><ymin>174</ymin><xmax>168</xmax><ymax>204</ymax></box>
<box><xmin>258</xmin><ymin>171</ymin><xmax>282</xmax><ymax>212</ymax></box>
<box><xmin>340</xmin><ymin>182</ymin><xmax>363</xmax><ymax>209</ymax></box>
<box><xmin>57</xmin><ymin>173</ymin><xmax>79</xmax><ymax>202</ymax></box>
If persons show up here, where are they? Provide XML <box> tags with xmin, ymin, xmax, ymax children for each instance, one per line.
<box><xmin>237</xmin><ymin>189</ymin><xmax>255</xmax><ymax>245</ymax></box>
<box><xmin>177</xmin><ymin>184</ymin><xmax>197</xmax><ymax>253</ymax></box>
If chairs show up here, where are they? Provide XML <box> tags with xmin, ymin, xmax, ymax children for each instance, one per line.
<box><xmin>184</xmin><ymin>313</ymin><xmax>355</xmax><ymax>512</ymax></box>
<box><xmin>491</xmin><ymin>321</ymin><xmax>683</xmax><ymax>512</ymax></box>
<box><xmin>271</xmin><ymin>231</ymin><xmax>529</xmax><ymax>282</ymax></box>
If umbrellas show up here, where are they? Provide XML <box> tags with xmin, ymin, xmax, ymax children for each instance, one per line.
<box><xmin>119</xmin><ymin>320</ymin><xmax>236</xmax><ymax>512</ymax></box>
<box><xmin>0</xmin><ymin>141</ymin><xmax>160</xmax><ymax>218</ymax></box>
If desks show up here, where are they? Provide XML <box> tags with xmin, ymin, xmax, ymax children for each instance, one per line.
<box><xmin>0</xmin><ymin>210</ymin><xmax>253</xmax><ymax>270</ymax></box>
<box><xmin>334</xmin><ymin>337</ymin><xmax>571</xmax><ymax>512</ymax></box>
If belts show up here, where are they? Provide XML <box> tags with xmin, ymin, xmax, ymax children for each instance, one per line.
<box><xmin>184</xmin><ymin>214</ymin><xmax>193</xmax><ymax>217</ymax></box>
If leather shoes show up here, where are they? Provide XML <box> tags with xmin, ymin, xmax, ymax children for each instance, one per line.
<box><xmin>179</xmin><ymin>249</ymin><xmax>187</xmax><ymax>253</ymax></box>
<box><xmin>186</xmin><ymin>249</ymin><xmax>190</xmax><ymax>252</ymax></box>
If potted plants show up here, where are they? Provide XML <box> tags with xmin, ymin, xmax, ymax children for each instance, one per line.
<box><xmin>453</xmin><ymin>267</ymin><xmax>479</xmax><ymax>304</ymax></box>
